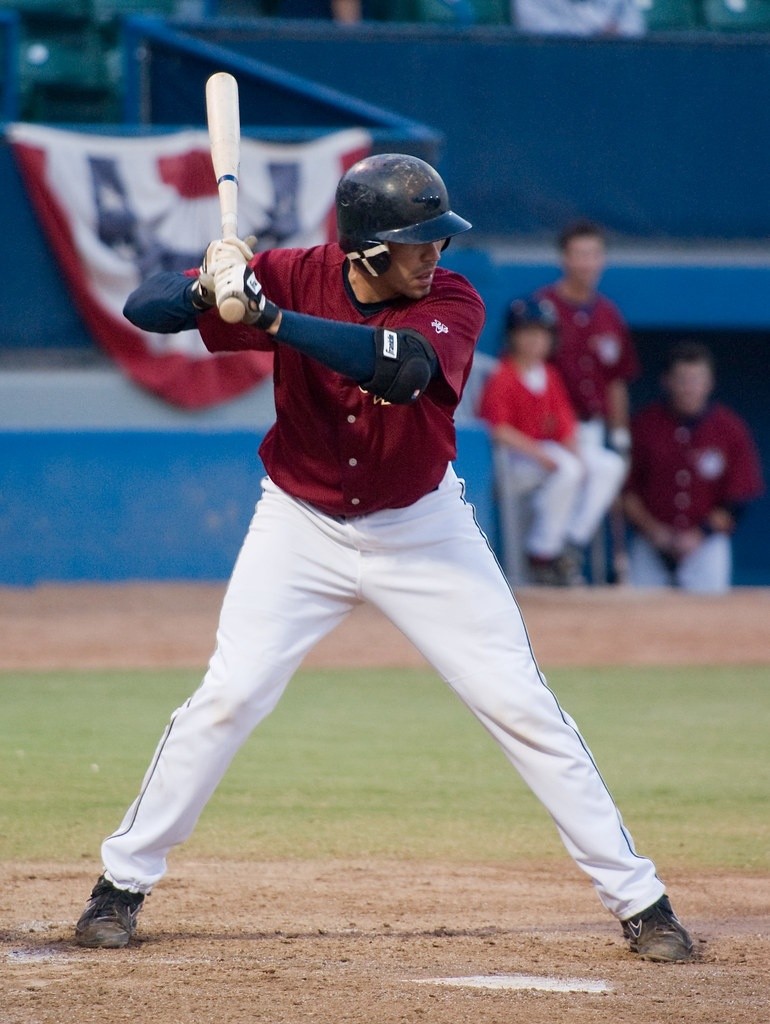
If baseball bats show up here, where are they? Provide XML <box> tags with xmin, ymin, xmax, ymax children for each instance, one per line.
<box><xmin>205</xmin><ymin>71</ymin><xmax>249</xmax><ymax>324</ymax></box>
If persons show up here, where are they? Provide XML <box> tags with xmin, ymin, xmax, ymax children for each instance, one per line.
<box><xmin>75</xmin><ymin>153</ymin><xmax>694</xmax><ymax>963</ymax></box>
<box><xmin>475</xmin><ymin>223</ymin><xmax>759</xmax><ymax>590</ymax></box>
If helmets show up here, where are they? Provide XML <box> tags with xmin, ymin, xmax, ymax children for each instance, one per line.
<box><xmin>335</xmin><ymin>153</ymin><xmax>473</xmax><ymax>276</ymax></box>
<box><xmin>506</xmin><ymin>299</ymin><xmax>558</xmax><ymax>330</ymax></box>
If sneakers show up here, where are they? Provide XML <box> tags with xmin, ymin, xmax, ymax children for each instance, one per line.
<box><xmin>76</xmin><ymin>875</ymin><xmax>151</xmax><ymax>948</ymax></box>
<box><xmin>622</xmin><ymin>894</ymin><xmax>692</xmax><ymax>961</ymax></box>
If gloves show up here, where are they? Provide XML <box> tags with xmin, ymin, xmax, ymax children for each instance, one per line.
<box><xmin>190</xmin><ymin>233</ymin><xmax>280</xmax><ymax>331</ymax></box>
